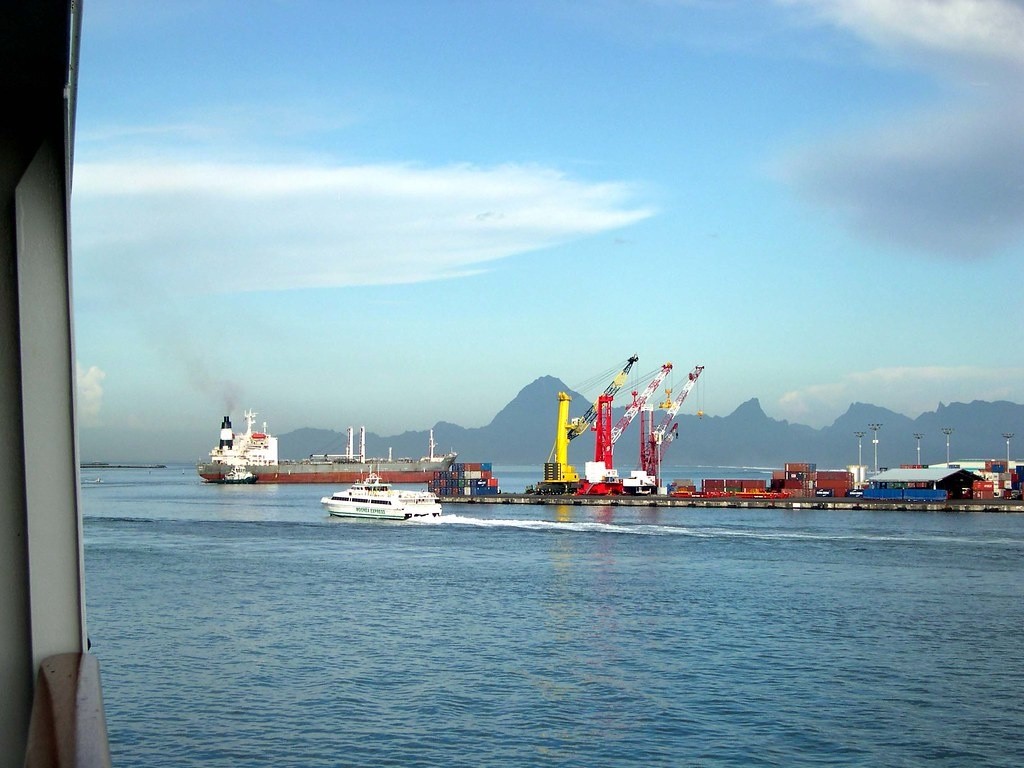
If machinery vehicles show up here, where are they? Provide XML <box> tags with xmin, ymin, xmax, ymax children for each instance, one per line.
<box><xmin>534</xmin><ymin>352</ymin><xmax>706</xmax><ymax>496</ymax></box>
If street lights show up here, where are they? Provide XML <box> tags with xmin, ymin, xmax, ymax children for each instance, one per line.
<box><xmin>1001</xmin><ymin>432</ymin><xmax>1016</xmax><ymax>474</ymax></box>
<box><xmin>868</xmin><ymin>423</ymin><xmax>884</xmax><ymax>476</ymax></box>
<box><xmin>912</xmin><ymin>432</ymin><xmax>925</xmax><ymax>465</ymax></box>
<box><xmin>853</xmin><ymin>431</ymin><xmax>867</xmax><ymax>482</ymax></box>
<box><xmin>941</xmin><ymin>426</ymin><xmax>955</xmax><ymax>469</ymax></box>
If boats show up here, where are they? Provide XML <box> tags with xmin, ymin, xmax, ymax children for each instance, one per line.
<box><xmin>194</xmin><ymin>407</ymin><xmax>459</xmax><ymax>485</ymax></box>
<box><xmin>320</xmin><ymin>462</ymin><xmax>442</xmax><ymax>521</ymax></box>
